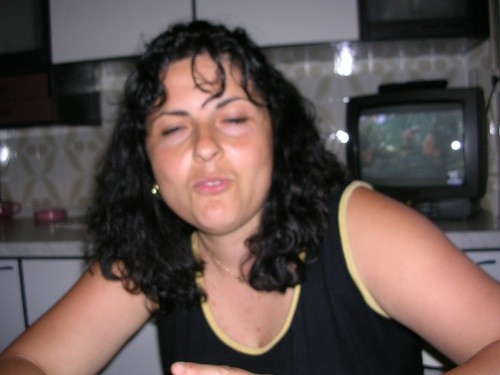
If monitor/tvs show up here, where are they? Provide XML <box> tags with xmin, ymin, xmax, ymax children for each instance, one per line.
<box><xmin>348</xmin><ymin>86</ymin><xmax>488</xmax><ymax>219</ymax></box>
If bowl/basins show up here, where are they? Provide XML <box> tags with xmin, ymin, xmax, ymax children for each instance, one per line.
<box><xmin>34</xmin><ymin>208</ymin><xmax>67</xmax><ymax>223</ymax></box>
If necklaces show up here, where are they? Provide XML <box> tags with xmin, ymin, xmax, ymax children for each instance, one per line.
<box><xmin>196</xmin><ymin>232</ymin><xmax>250</xmax><ymax>284</ymax></box>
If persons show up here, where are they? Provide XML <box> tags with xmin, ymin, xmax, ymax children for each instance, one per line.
<box><xmin>0</xmin><ymin>20</ymin><xmax>500</xmax><ymax>375</ymax></box>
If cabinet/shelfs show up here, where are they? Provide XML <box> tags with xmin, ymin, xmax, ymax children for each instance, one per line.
<box><xmin>0</xmin><ymin>228</ymin><xmax>500</xmax><ymax>375</ymax></box>
<box><xmin>51</xmin><ymin>0</ymin><xmax>360</xmax><ymax>68</ymax></box>
